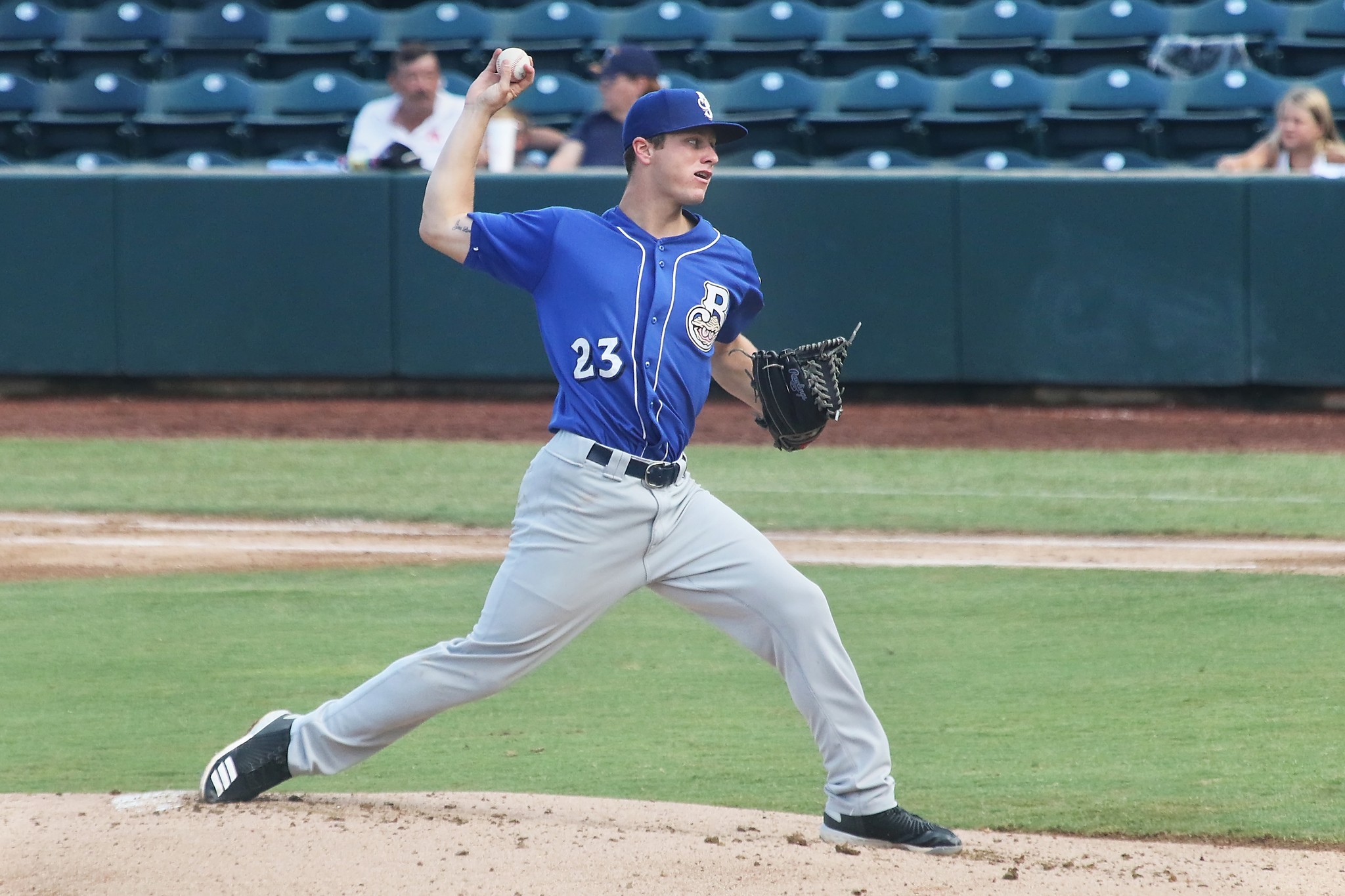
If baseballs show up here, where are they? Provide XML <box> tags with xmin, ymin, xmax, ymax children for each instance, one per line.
<box><xmin>495</xmin><ymin>46</ymin><xmax>534</xmax><ymax>86</ymax></box>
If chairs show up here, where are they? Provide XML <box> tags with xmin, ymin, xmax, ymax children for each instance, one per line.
<box><xmin>0</xmin><ymin>0</ymin><xmax>1345</xmax><ymax>170</ymax></box>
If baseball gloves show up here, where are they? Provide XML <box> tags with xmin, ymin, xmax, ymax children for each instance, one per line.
<box><xmin>744</xmin><ymin>335</ymin><xmax>854</xmax><ymax>454</ymax></box>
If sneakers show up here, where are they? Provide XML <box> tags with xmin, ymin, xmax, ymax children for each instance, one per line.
<box><xmin>199</xmin><ymin>710</ymin><xmax>300</xmax><ymax>805</ymax></box>
<box><xmin>820</xmin><ymin>804</ymin><xmax>963</xmax><ymax>856</ymax></box>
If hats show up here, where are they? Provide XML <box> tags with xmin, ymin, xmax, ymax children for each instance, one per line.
<box><xmin>623</xmin><ymin>89</ymin><xmax>748</xmax><ymax>150</ymax></box>
<box><xmin>588</xmin><ymin>46</ymin><xmax>659</xmax><ymax>78</ymax></box>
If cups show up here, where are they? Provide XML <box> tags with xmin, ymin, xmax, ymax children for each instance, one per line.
<box><xmin>486</xmin><ymin>118</ymin><xmax>516</xmax><ymax>174</ymax></box>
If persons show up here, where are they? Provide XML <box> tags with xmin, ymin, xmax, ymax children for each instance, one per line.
<box><xmin>346</xmin><ymin>40</ymin><xmax>584</xmax><ymax>173</ymax></box>
<box><xmin>569</xmin><ymin>44</ymin><xmax>662</xmax><ymax>167</ymax></box>
<box><xmin>201</xmin><ymin>48</ymin><xmax>964</xmax><ymax>856</ymax></box>
<box><xmin>1216</xmin><ymin>85</ymin><xmax>1345</xmax><ymax>176</ymax></box>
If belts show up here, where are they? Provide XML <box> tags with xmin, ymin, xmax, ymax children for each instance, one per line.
<box><xmin>586</xmin><ymin>443</ymin><xmax>687</xmax><ymax>488</ymax></box>
